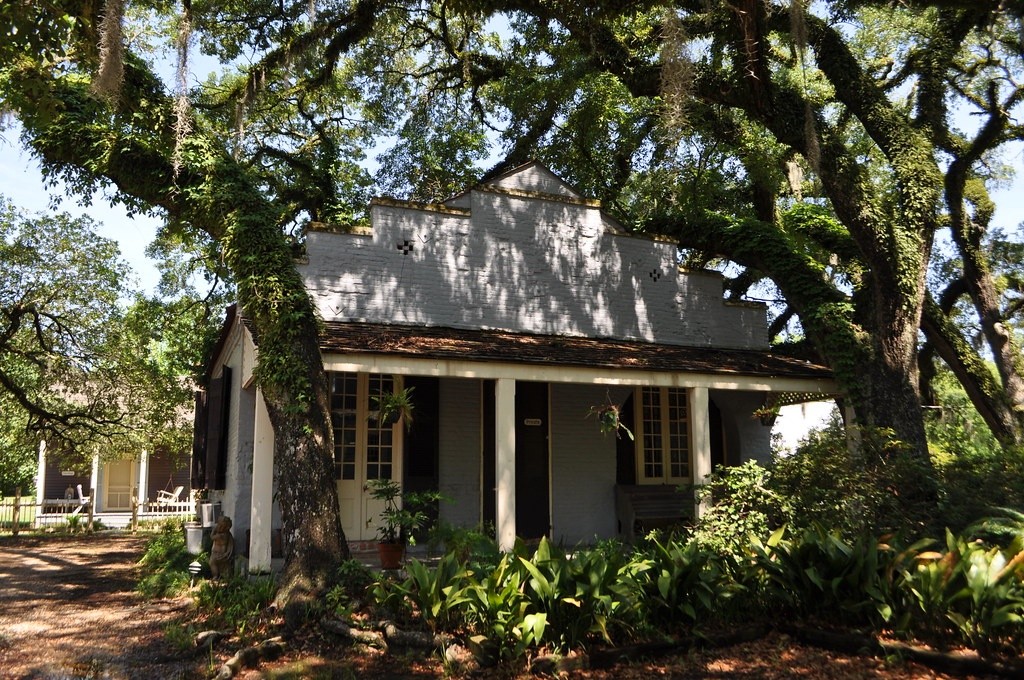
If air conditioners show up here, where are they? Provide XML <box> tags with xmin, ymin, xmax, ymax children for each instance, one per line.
<box><xmin>200</xmin><ymin>503</ymin><xmax>221</xmax><ymax>527</ymax></box>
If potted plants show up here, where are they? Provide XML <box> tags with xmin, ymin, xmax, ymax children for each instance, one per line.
<box><xmin>364</xmin><ymin>375</ymin><xmax>415</xmax><ymax>434</ymax></box>
<box><xmin>752</xmin><ymin>405</ymin><xmax>782</xmax><ymax>427</ymax></box>
<box><xmin>582</xmin><ymin>402</ymin><xmax>635</xmax><ymax>443</ymax></box>
<box><xmin>362</xmin><ymin>478</ymin><xmax>454</xmax><ymax>568</ymax></box>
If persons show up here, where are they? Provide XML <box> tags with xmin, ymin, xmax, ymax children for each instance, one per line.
<box><xmin>64</xmin><ymin>484</ymin><xmax>74</xmax><ymax>512</ymax></box>
<box><xmin>209</xmin><ymin>516</ymin><xmax>232</xmax><ymax>581</ymax></box>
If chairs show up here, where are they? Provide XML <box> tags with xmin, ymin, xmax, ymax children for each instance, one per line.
<box><xmin>157</xmin><ymin>486</ymin><xmax>184</xmax><ymax>511</ymax></box>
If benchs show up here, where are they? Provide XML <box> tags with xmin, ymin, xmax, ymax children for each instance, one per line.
<box><xmin>613</xmin><ymin>483</ymin><xmax>693</xmax><ymax>540</ymax></box>
